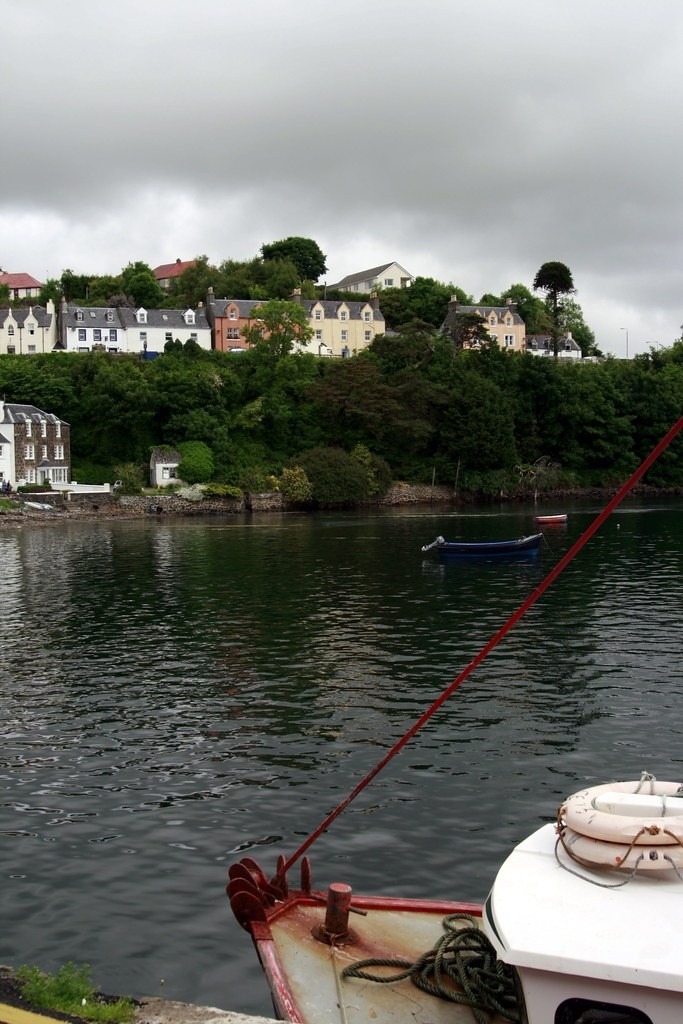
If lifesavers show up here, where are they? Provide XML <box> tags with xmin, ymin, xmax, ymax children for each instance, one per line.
<box><xmin>560</xmin><ymin>825</ymin><xmax>683</xmax><ymax>871</ymax></box>
<box><xmin>562</xmin><ymin>780</ymin><xmax>683</xmax><ymax>846</ymax></box>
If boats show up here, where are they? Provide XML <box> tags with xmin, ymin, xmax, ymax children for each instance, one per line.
<box><xmin>224</xmin><ymin>774</ymin><xmax>683</xmax><ymax>1024</ymax></box>
<box><xmin>421</xmin><ymin>532</ymin><xmax>543</xmax><ymax>558</ymax></box>
<box><xmin>532</xmin><ymin>512</ymin><xmax>569</xmax><ymax>524</ymax></box>
<box><xmin>50</xmin><ymin>482</ymin><xmax>110</xmax><ymax>494</ymax></box>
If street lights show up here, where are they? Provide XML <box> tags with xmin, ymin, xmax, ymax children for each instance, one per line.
<box><xmin>620</xmin><ymin>327</ymin><xmax>629</xmax><ymax>365</ymax></box>
<box><xmin>647</xmin><ymin>339</ymin><xmax>659</xmax><ymax>353</ymax></box>
